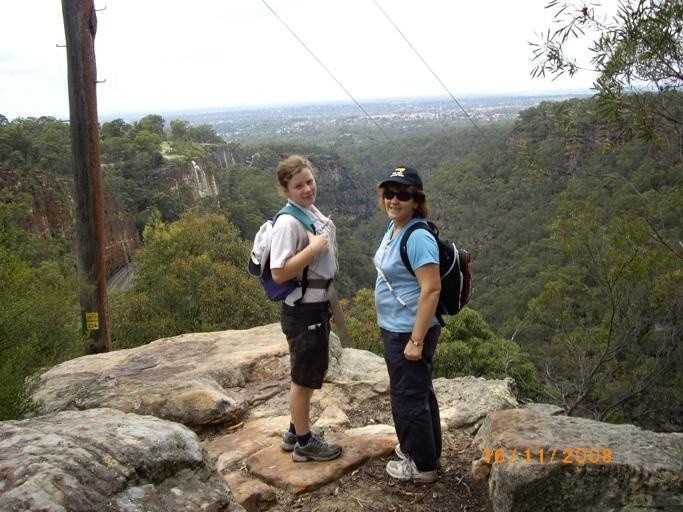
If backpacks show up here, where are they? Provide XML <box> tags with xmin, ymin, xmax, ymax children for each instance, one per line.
<box><xmin>256</xmin><ymin>208</ymin><xmax>318</xmax><ymax>305</ymax></box>
<box><xmin>399</xmin><ymin>220</ymin><xmax>475</xmax><ymax>316</ymax></box>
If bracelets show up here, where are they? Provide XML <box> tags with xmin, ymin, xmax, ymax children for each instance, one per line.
<box><xmin>408</xmin><ymin>336</ymin><xmax>424</xmax><ymax>347</ymax></box>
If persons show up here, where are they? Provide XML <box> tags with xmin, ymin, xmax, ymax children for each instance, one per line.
<box><xmin>266</xmin><ymin>150</ymin><xmax>344</xmax><ymax>466</ymax></box>
<box><xmin>370</xmin><ymin>163</ymin><xmax>446</xmax><ymax>487</ymax></box>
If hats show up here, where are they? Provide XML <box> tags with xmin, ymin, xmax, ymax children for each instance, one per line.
<box><xmin>379</xmin><ymin>166</ymin><xmax>422</xmax><ymax>189</ymax></box>
<box><xmin>247</xmin><ymin>219</ymin><xmax>272</xmax><ymax>278</ymax></box>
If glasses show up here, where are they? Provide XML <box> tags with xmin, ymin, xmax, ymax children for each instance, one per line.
<box><xmin>383</xmin><ymin>189</ymin><xmax>418</xmax><ymax>201</ymax></box>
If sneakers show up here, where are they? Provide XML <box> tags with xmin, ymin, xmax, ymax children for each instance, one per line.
<box><xmin>292</xmin><ymin>437</ymin><xmax>342</xmax><ymax>462</ymax></box>
<box><xmin>393</xmin><ymin>443</ymin><xmax>408</xmax><ymax>461</ymax></box>
<box><xmin>386</xmin><ymin>456</ymin><xmax>438</xmax><ymax>482</ymax></box>
<box><xmin>280</xmin><ymin>429</ymin><xmax>324</xmax><ymax>450</ymax></box>
